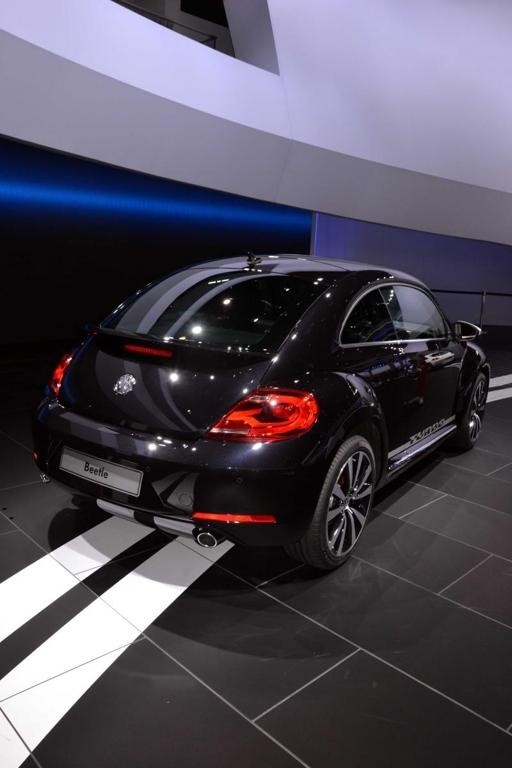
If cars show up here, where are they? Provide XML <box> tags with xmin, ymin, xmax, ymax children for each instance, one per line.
<box><xmin>33</xmin><ymin>246</ymin><xmax>493</xmax><ymax>570</ymax></box>
